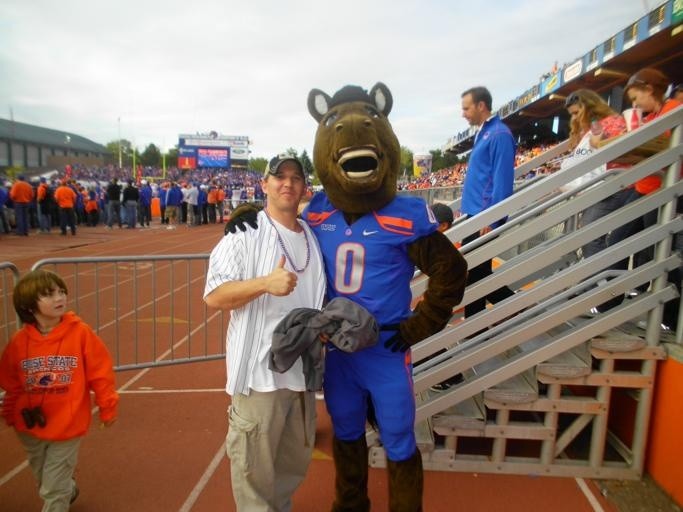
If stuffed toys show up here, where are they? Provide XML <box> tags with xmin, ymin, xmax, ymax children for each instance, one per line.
<box><xmin>222</xmin><ymin>79</ymin><xmax>467</xmax><ymax>511</ymax></box>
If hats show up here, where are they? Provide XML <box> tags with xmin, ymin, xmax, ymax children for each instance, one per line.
<box><xmin>162</xmin><ymin>181</ymin><xmax>206</xmax><ymax>189</ymax></box>
<box><xmin>263</xmin><ymin>151</ymin><xmax>306</xmax><ymax>182</ymax></box>
<box><xmin>18</xmin><ymin>174</ymin><xmax>72</xmax><ymax>184</ymax></box>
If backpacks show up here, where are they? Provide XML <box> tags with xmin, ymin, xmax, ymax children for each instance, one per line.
<box><xmin>40</xmin><ymin>186</ymin><xmax>53</xmax><ymax>214</ymax></box>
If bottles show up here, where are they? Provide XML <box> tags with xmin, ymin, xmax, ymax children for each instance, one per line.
<box><xmin>591</xmin><ymin>117</ymin><xmax>603</xmax><ymax>136</ymax></box>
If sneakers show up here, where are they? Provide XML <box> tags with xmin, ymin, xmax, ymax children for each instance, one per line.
<box><xmin>104</xmin><ymin>223</ymin><xmax>150</xmax><ymax>230</ymax></box>
<box><xmin>165</xmin><ymin>223</ymin><xmax>177</xmax><ymax>230</ymax></box>
<box><xmin>429</xmin><ymin>376</ymin><xmax>466</xmax><ymax>392</ymax></box>
<box><xmin>13</xmin><ymin>228</ymin><xmax>51</xmax><ymax>235</ymax></box>
<box><xmin>636</xmin><ymin>317</ymin><xmax>674</xmax><ymax>334</ymax></box>
<box><xmin>579</xmin><ymin>306</ymin><xmax>601</xmax><ymax>320</ymax></box>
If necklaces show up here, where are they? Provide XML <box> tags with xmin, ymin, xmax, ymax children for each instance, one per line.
<box><xmin>265</xmin><ymin>207</ymin><xmax>308</xmax><ymax>273</ymax></box>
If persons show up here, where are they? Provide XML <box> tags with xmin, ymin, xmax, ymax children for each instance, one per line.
<box><xmin>395</xmin><ymin>163</ymin><xmax>466</xmax><ymax>217</ymax></box>
<box><xmin>459</xmin><ymin>85</ymin><xmax>522</xmax><ymax>343</ymax></box>
<box><xmin>515</xmin><ymin>142</ymin><xmax>561</xmax><ymax>181</ymax></box>
<box><xmin>550</xmin><ymin>64</ymin><xmax>682</xmax><ymax>338</ymax></box>
<box><xmin>405</xmin><ymin>202</ymin><xmax>464</xmax><ymax>393</ymax></box>
<box><xmin>54</xmin><ymin>181</ymin><xmax>77</xmax><ymax>237</ymax></box>
<box><xmin>10</xmin><ymin>174</ymin><xmax>34</xmax><ymax>239</ymax></box>
<box><xmin>0</xmin><ymin>269</ymin><xmax>118</xmax><ymax>512</ymax></box>
<box><xmin>306</xmin><ymin>183</ymin><xmax>320</xmax><ymax>197</ymax></box>
<box><xmin>200</xmin><ymin>152</ymin><xmax>332</xmax><ymax>512</ymax></box>
<box><xmin>1</xmin><ymin>167</ymin><xmax>267</xmax><ymax>233</ymax></box>
